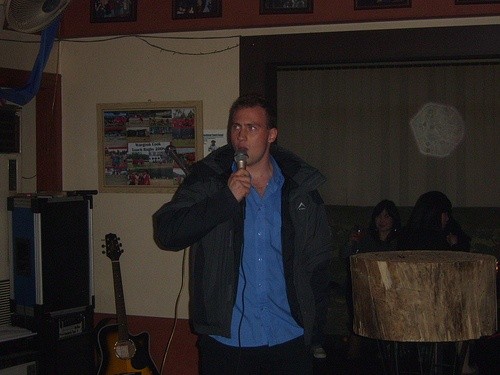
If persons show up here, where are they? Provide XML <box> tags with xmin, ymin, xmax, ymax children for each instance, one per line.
<box><xmin>151</xmin><ymin>93</ymin><xmax>338</xmax><ymax>375</ymax></box>
<box><xmin>359</xmin><ymin>199</ymin><xmax>403</xmax><ymax>253</ymax></box>
<box><xmin>396</xmin><ymin>191</ymin><xmax>473</xmax><ymax>252</ymax></box>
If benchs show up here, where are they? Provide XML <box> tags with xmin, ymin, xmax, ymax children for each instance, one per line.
<box><xmin>323</xmin><ymin>204</ymin><xmax>500</xmax><ymax>343</ymax></box>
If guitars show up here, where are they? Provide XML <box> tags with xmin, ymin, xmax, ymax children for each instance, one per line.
<box><xmin>97</xmin><ymin>232</ymin><xmax>161</xmax><ymax>375</ymax></box>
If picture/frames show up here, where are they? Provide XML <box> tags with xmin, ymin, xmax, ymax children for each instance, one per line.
<box><xmin>259</xmin><ymin>0</ymin><xmax>313</xmax><ymax>14</ymax></box>
<box><xmin>89</xmin><ymin>0</ymin><xmax>137</xmax><ymax>23</ymax></box>
<box><xmin>170</xmin><ymin>0</ymin><xmax>223</xmax><ymax>20</ymax></box>
<box><xmin>95</xmin><ymin>99</ymin><xmax>204</xmax><ymax>194</ymax></box>
<box><xmin>354</xmin><ymin>0</ymin><xmax>412</xmax><ymax>10</ymax></box>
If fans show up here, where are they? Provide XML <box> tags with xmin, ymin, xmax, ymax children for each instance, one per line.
<box><xmin>4</xmin><ymin>0</ymin><xmax>71</xmax><ymax>34</ymax></box>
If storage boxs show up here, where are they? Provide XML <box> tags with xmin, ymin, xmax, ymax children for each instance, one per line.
<box><xmin>5</xmin><ymin>193</ymin><xmax>101</xmax><ymax>316</ymax></box>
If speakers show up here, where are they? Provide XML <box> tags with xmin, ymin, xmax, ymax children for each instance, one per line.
<box><xmin>12</xmin><ymin>310</ymin><xmax>97</xmax><ymax>374</ymax></box>
<box><xmin>8</xmin><ymin>192</ymin><xmax>96</xmax><ymax>314</ymax></box>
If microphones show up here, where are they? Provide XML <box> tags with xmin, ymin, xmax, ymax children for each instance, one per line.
<box><xmin>233</xmin><ymin>147</ymin><xmax>249</xmax><ymax>220</ymax></box>
<box><xmin>165</xmin><ymin>145</ymin><xmax>189</xmax><ymax>175</ymax></box>
